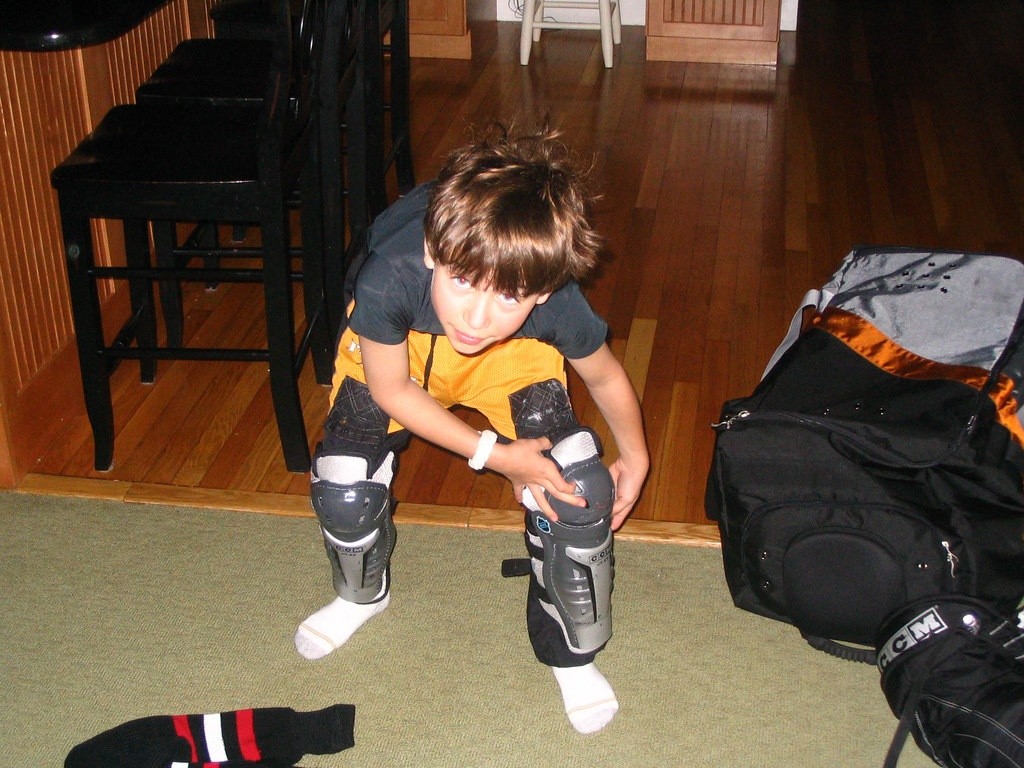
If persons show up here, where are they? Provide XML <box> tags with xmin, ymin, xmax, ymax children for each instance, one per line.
<box><xmin>296</xmin><ymin>110</ymin><xmax>650</xmax><ymax>733</ymax></box>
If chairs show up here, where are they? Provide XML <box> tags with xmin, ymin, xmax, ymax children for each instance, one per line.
<box><xmin>50</xmin><ymin>0</ymin><xmax>417</xmax><ymax>474</ymax></box>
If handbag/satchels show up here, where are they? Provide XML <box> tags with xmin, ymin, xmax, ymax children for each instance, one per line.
<box><xmin>704</xmin><ymin>247</ymin><xmax>1024</xmax><ymax>768</ymax></box>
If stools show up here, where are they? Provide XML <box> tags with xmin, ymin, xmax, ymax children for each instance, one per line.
<box><xmin>519</xmin><ymin>0</ymin><xmax>622</xmax><ymax>68</ymax></box>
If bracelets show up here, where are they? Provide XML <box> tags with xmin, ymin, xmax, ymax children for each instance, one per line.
<box><xmin>468</xmin><ymin>430</ymin><xmax>498</xmax><ymax>471</ymax></box>
